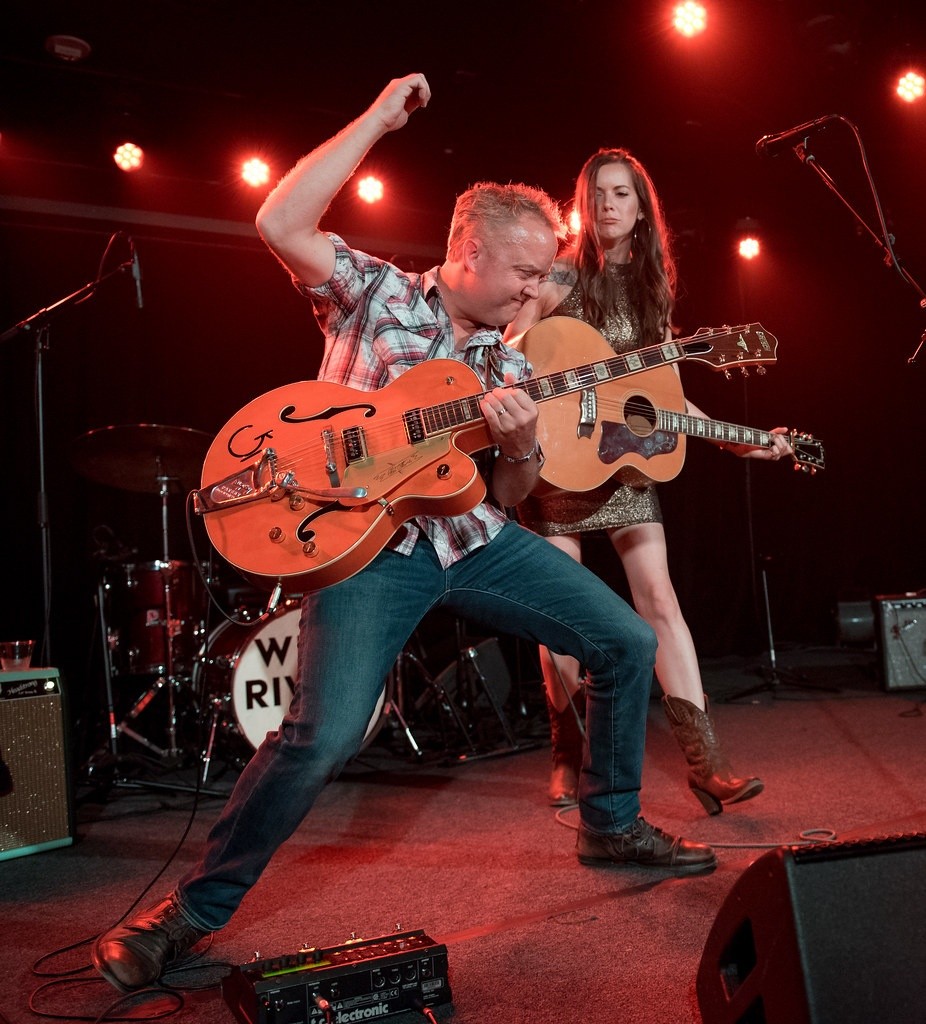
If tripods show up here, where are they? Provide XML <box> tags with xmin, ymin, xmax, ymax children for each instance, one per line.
<box><xmin>73</xmin><ymin>449</ymin><xmax>436</xmax><ymax>811</ymax></box>
<box><xmin>716</xmin><ymin>568</ymin><xmax>842</xmax><ymax>702</ymax></box>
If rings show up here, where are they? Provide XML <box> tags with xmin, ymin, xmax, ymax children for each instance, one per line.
<box><xmin>498</xmin><ymin>408</ymin><xmax>508</xmax><ymax>417</ymax></box>
<box><xmin>771</xmin><ymin>452</ymin><xmax>777</xmax><ymax>457</ymax></box>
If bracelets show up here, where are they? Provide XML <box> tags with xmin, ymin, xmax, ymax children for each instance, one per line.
<box><xmin>498</xmin><ymin>445</ymin><xmax>536</xmax><ymax>464</ymax></box>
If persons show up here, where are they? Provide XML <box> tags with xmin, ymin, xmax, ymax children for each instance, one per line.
<box><xmin>499</xmin><ymin>147</ymin><xmax>795</xmax><ymax>816</ymax></box>
<box><xmin>90</xmin><ymin>69</ymin><xmax>719</xmax><ymax>1003</ymax></box>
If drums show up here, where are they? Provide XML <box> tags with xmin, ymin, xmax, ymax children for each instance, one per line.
<box><xmin>191</xmin><ymin>593</ymin><xmax>396</xmax><ymax>767</ymax></box>
<box><xmin>100</xmin><ymin>557</ymin><xmax>219</xmax><ymax>677</ymax></box>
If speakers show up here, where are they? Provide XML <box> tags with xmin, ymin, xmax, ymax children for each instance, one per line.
<box><xmin>694</xmin><ymin>830</ymin><xmax>926</xmax><ymax>1024</ymax></box>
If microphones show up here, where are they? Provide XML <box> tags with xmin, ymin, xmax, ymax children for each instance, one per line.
<box><xmin>126</xmin><ymin>235</ymin><xmax>143</xmax><ymax>308</ymax></box>
<box><xmin>755</xmin><ymin>114</ymin><xmax>838</xmax><ymax>162</ymax></box>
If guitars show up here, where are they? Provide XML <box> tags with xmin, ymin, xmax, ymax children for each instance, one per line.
<box><xmin>189</xmin><ymin>320</ymin><xmax>779</xmax><ymax>592</ymax></box>
<box><xmin>511</xmin><ymin>314</ymin><xmax>823</xmax><ymax>496</ymax></box>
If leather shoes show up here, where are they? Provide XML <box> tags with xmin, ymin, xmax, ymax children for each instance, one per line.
<box><xmin>575</xmin><ymin>816</ymin><xmax>718</xmax><ymax>871</ymax></box>
<box><xmin>91</xmin><ymin>893</ymin><xmax>204</xmax><ymax>996</ymax></box>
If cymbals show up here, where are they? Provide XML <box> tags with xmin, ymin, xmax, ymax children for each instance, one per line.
<box><xmin>62</xmin><ymin>421</ymin><xmax>215</xmax><ymax>494</ymax></box>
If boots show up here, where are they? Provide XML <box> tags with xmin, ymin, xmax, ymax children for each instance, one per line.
<box><xmin>546</xmin><ymin>683</ymin><xmax>584</xmax><ymax>806</ymax></box>
<box><xmin>662</xmin><ymin>693</ymin><xmax>765</xmax><ymax>816</ymax></box>
